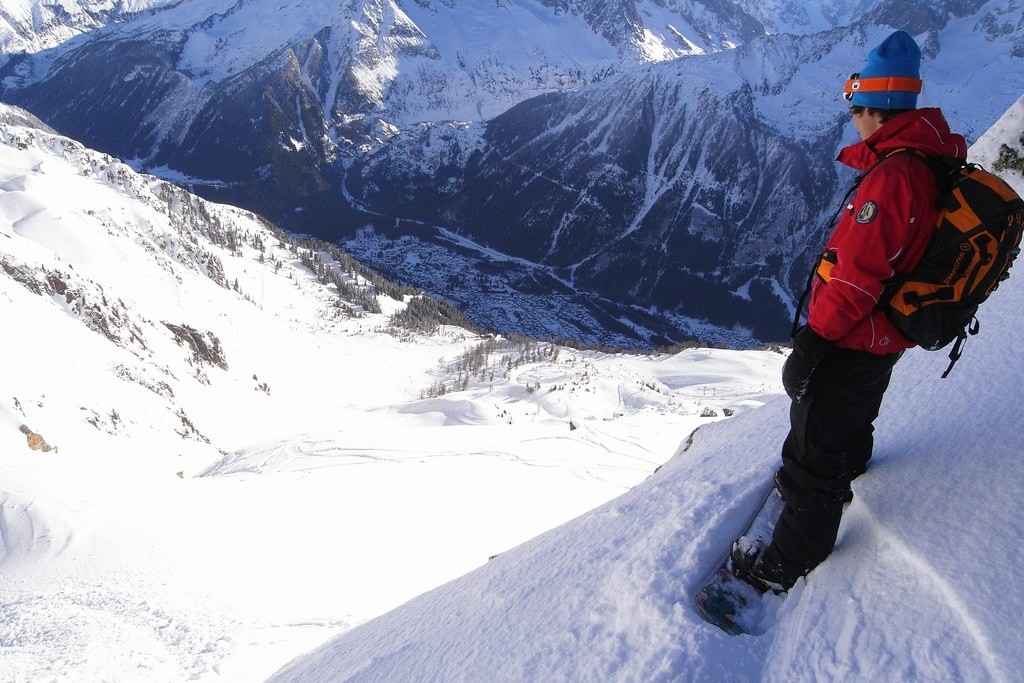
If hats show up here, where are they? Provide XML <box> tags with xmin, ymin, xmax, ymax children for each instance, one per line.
<box><xmin>849</xmin><ymin>30</ymin><xmax>922</xmax><ymax>112</ymax></box>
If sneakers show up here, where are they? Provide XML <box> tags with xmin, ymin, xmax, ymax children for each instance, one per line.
<box><xmin>729</xmin><ymin>536</ymin><xmax>792</xmax><ymax>592</ymax></box>
<box><xmin>772</xmin><ymin>469</ymin><xmax>789</xmax><ymax>498</ymax></box>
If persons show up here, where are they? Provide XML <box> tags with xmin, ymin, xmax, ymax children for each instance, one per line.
<box><xmin>733</xmin><ymin>31</ymin><xmax>1023</xmax><ymax>595</ymax></box>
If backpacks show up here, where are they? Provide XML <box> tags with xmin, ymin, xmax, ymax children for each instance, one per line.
<box><xmin>877</xmin><ymin>148</ymin><xmax>1022</xmax><ymax>351</ymax></box>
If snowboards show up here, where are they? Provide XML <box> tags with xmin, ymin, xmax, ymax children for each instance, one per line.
<box><xmin>692</xmin><ymin>485</ymin><xmax>791</xmax><ymax>638</ymax></box>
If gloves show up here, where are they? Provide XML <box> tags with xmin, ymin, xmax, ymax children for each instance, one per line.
<box><xmin>782</xmin><ymin>323</ymin><xmax>836</xmax><ymax>403</ymax></box>
